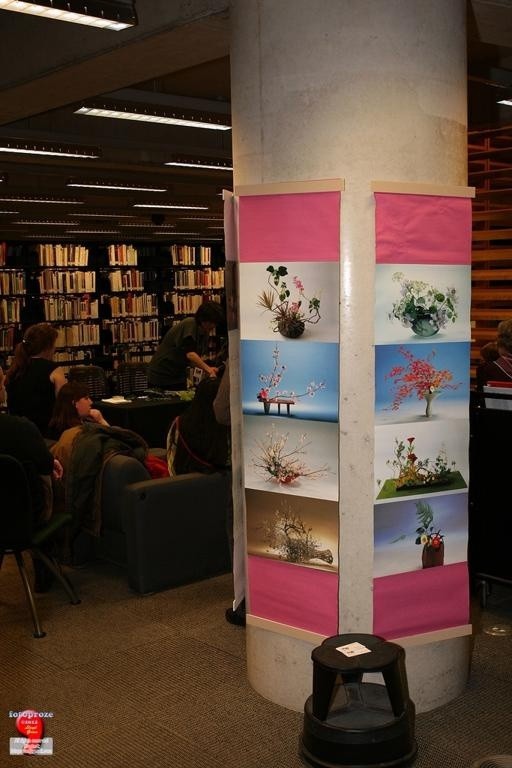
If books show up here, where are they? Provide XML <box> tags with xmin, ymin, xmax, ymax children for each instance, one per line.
<box><xmin>209</xmin><ymin>332</ymin><xmax>223</xmax><ymax>361</ymax></box>
<box><xmin>56</xmin><ymin>349</ymin><xmax>95</xmax><ymax>366</ymax></box>
<box><xmin>91</xmin><ymin>241</ymin><xmax>226</xmax><ymax>267</ymax></box>
<box><xmin>0</xmin><ymin>269</ymin><xmax>228</xmax><ymax>295</ymax></box>
<box><xmin>70</xmin><ymin>323</ymin><xmax>100</xmax><ymax>346</ymax></box>
<box><xmin>102</xmin><ymin>321</ymin><xmax>159</xmax><ymax>343</ymax></box>
<box><xmin>0</xmin><ymin>241</ymin><xmax>90</xmax><ymax>268</ymax></box>
<box><xmin>103</xmin><ymin>344</ymin><xmax>155</xmax><ymax>368</ymax></box>
<box><xmin>1</xmin><ymin>295</ymin><xmax>156</xmax><ymax>322</ymax></box>
<box><xmin>172</xmin><ymin>295</ymin><xmax>223</xmax><ymax>315</ymax></box>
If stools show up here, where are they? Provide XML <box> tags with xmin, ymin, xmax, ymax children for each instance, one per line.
<box><xmin>298</xmin><ymin>632</ymin><xmax>418</xmax><ymax>768</ymax></box>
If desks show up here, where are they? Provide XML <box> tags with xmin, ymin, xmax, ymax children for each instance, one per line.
<box><xmin>92</xmin><ymin>392</ymin><xmax>198</xmax><ymax>448</ymax></box>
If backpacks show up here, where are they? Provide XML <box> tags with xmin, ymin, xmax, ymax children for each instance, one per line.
<box><xmin>166</xmin><ymin>377</ymin><xmax>231</xmax><ymax>476</ymax></box>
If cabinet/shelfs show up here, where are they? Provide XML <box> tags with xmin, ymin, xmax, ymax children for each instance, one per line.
<box><xmin>1</xmin><ymin>234</ymin><xmax>230</xmax><ymax>409</ymax></box>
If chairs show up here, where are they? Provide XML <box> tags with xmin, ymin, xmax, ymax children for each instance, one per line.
<box><xmin>69</xmin><ymin>366</ymin><xmax>110</xmax><ymax>399</ymax></box>
<box><xmin>115</xmin><ymin>363</ymin><xmax>148</xmax><ymax>395</ymax></box>
<box><xmin>0</xmin><ymin>453</ymin><xmax>82</xmax><ymax>638</ymax></box>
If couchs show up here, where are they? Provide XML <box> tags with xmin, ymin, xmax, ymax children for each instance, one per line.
<box><xmin>86</xmin><ymin>449</ymin><xmax>233</xmax><ymax>597</ymax></box>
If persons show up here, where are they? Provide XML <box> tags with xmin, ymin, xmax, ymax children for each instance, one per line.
<box><xmin>49</xmin><ymin>379</ymin><xmax>170</xmax><ymax>479</ymax></box>
<box><xmin>476</xmin><ymin>340</ymin><xmax>500</xmax><ymax>386</ymax></box>
<box><xmin>479</xmin><ymin>320</ymin><xmax>512</xmax><ymax>383</ymax></box>
<box><xmin>0</xmin><ymin>369</ymin><xmax>65</xmax><ymax>594</ymax></box>
<box><xmin>147</xmin><ymin>302</ymin><xmax>226</xmax><ymax>392</ymax></box>
<box><xmin>4</xmin><ymin>322</ymin><xmax>69</xmax><ymax>440</ymax></box>
